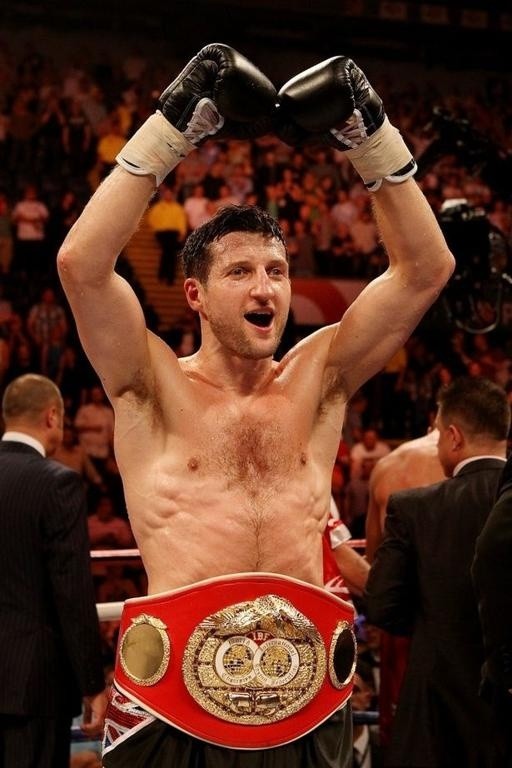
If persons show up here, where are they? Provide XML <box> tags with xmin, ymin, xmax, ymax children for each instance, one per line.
<box><xmin>1</xmin><ymin>0</ymin><xmax>512</xmax><ymax>768</ymax></box>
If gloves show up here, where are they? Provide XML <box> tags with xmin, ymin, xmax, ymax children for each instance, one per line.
<box><xmin>115</xmin><ymin>44</ymin><xmax>277</xmax><ymax>189</ymax></box>
<box><xmin>277</xmin><ymin>54</ymin><xmax>420</xmax><ymax>192</ymax></box>
